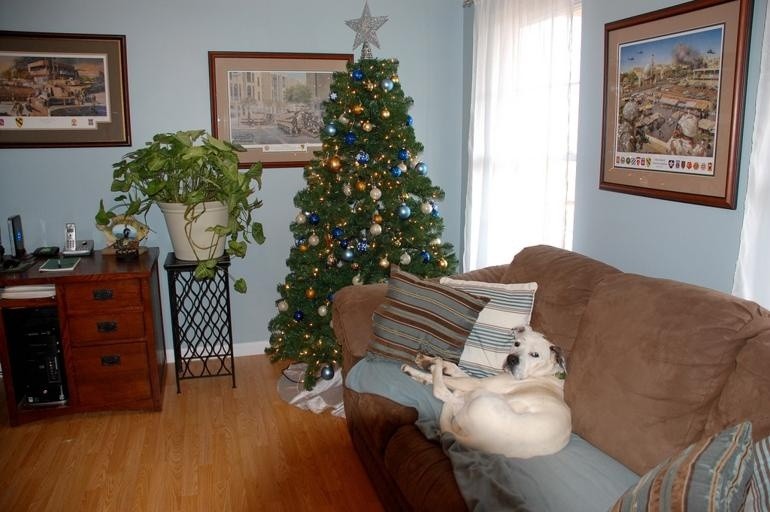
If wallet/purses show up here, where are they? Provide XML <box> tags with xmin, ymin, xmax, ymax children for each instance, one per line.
<box><xmin>33</xmin><ymin>246</ymin><xmax>59</xmax><ymax>258</ymax></box>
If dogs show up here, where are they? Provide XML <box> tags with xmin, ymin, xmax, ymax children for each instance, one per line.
<box><xmin>401</xmin><ymin>326</ymin><xmax>572</xmax><ymax>459</ymax></box>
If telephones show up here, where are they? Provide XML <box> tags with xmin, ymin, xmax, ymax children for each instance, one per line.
<box><xmin>61</xmin><ymin>222</ymin><xmax>94</xmax><ymax>258</ymax></box>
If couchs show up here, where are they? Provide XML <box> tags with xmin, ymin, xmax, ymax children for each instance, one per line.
<box><xmin>331</xmin><ymin>243</ymin><xmax>770</xmax><ymax>512</ymax></box>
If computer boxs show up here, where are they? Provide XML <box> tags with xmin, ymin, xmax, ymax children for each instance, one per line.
<box><xmin>8</xmin><ymin>310</ymin><xmax>67</xmax><ymax>406</ymax></box>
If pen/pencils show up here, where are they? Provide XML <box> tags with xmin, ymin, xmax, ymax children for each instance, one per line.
<box><xmin>58</xmin><ymin>260</ymin><xmax>61</xmax><ymax>268</ymax></box>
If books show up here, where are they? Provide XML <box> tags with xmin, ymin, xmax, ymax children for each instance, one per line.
<box><xmin>38</xmin><ymin>258</ymin><xmax>81</xmax><ymax>272</ymax></box>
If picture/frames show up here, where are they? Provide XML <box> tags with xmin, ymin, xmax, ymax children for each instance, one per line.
<box><xmin>1</xmin><ymin>30</ymin><xmax>132</xmax><ymax>149</ymax></box>
<box><xmin>597</xmin><ymin>0</ymin><xmax>756</xmax><ymax>212</ymax></box>
<box><xmin>207</xmin><ymin>50</ymin><xmax>355</xmax><ymax>168</ymax></box>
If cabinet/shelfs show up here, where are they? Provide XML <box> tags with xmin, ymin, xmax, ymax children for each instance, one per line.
<box><xmin>1</xmin><ymin>246</ymin><xmax>168</xmax><ymax>429</ymax></box>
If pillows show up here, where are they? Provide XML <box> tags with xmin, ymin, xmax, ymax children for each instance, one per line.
<box><xmin>614</xmin><ymin>420</ymin><xmax>770</xmax><ymax>510</ymax></box>
<box><xmin>366</xmin><ymin>262</ymin><xmax>539</xmax><ymax>380</ymax></box>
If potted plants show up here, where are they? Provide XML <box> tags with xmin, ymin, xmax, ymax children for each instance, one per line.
<box><xmin>94</xmin><ymin>129</ymin><xmax>266</xmax><ymax>294</ymax></box>
